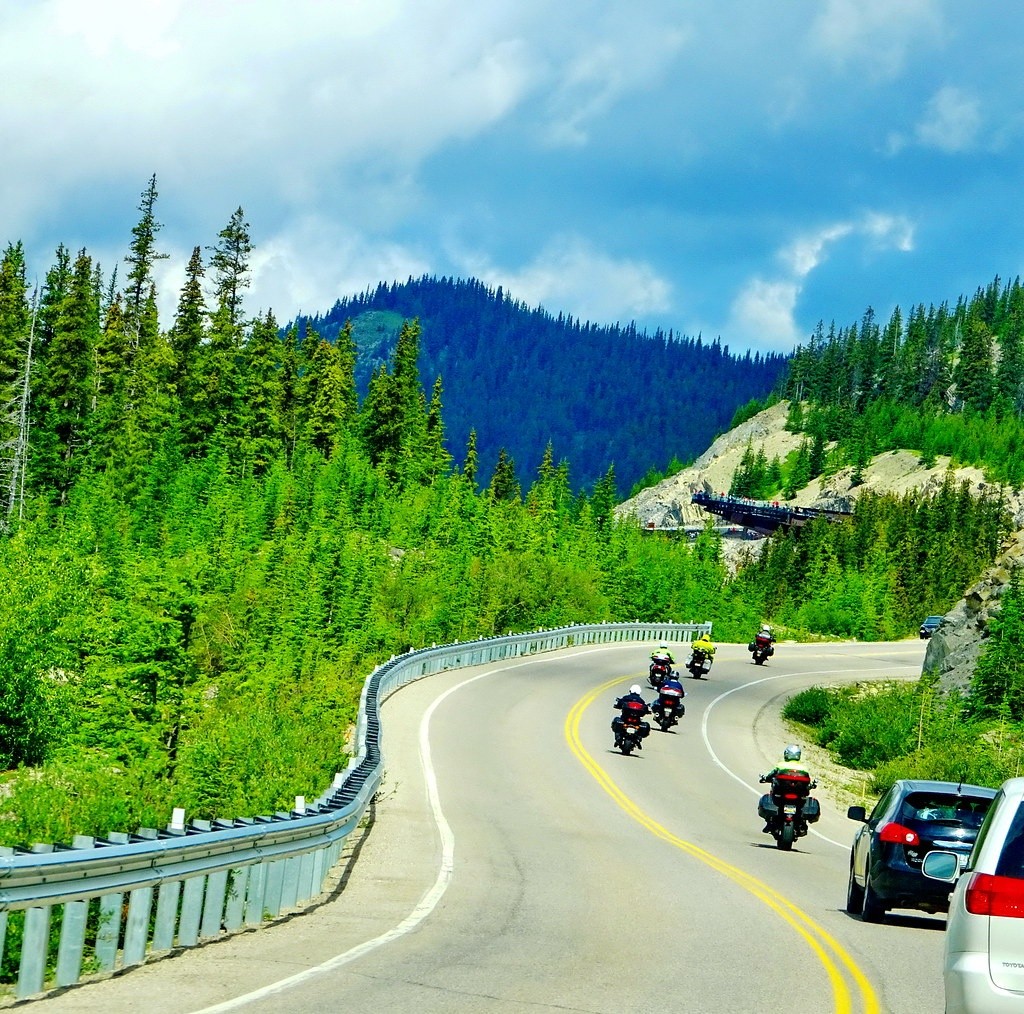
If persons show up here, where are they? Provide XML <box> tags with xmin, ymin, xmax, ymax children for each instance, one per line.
<box><xmin>611</xmin><ymin>685</ymin><xmax>650</xmax><ymax>750</ymax></box>
<box><xmin>651</xmin><ymin>671</ymin><xmax>685</xmax><ymax>721</ymax></box>
<box><xmin>758</xmin><ymin>745</ymin><xmax>820</xmax><ymax>833</ymax></box>
<box><xmin>749</xmin><ymin>625</ymin><xmax>774</xmax><ymax>660</ymax></box>
<box><xmin>690</xmin><ymin>635</ymin><xmax>715</xmax><ymax>671</ymax></box>
<box><xmin>649</xmin><ymin>641</ymin><xmax>676</xmax><ymax>683</ymax></box>
<box><xmin>916</xmin><ymin>802</ymin><xmax>988</xmax><ymax>822</ymax></box>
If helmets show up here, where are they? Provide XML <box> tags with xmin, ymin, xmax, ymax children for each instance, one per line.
<box><xmin>660</xmin><ymin>640</ymin><xmax>667</xmax><ymax>648</ymax></box>
<box><xmin>702</xmin><ymin>635</ymin><xmax>710</xmax><ymax>642</ymax></box>
<box><xmin>629</xmin><ymin>685</ymin><xmax>641</xmax><ymax>694</ymax></box>
<box><xmin>670</xmin><ymin>671</ymin><xmax>679</xmax><ymax>679</ymax></box>
<box><xmin>784</xmin><ymin>744</ymin><xmax>801</xmax><ymax>762</ymax></box>
<box><xmin>763</xmin><ymin>625</ymin><xmax>769</xmax><ymax>630</ymax></box>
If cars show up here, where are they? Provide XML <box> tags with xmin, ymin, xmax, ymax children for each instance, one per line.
<box><xmin>921</xmin><ymin>777</ymin><xmax>1024</xmax><ymax>1014</ymax></box>
<box><xmin>846</xmin><ymin>779</ymin><xmax>998</xmax><ymax>924</ymax></box>
<box><xmin>919</xmin><ymin>616</ymin><xmax>943</xmax><ymax>639</ymax></box>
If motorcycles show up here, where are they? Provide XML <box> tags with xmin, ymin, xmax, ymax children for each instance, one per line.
<box><xmin>610</xmin><ymin>697</ymin><xmax>652</xmax><ymax>757</ymax></box>
<box><xmin>685</xmin><ymin>641</ymin><xmax>717</xmax><ymax>680</ymax></box>
<box><xmin>649</xmin><ymin>685</ymin><xmax>687</xmax><ymax>732</ymax></box>
<box><xmin>748</xmin><ymin>630</ymin><xmax>777</xmax><ymax>666</ymax></box>
<box><xmin>647</xmin><ymin>652</ymin><xmax>675</xmax><ymax>690</ymax></box>
<box><xmin>756</xmin><ymin>772</ymin><xmax>821</xmax><ymax>851</ymax></box>
